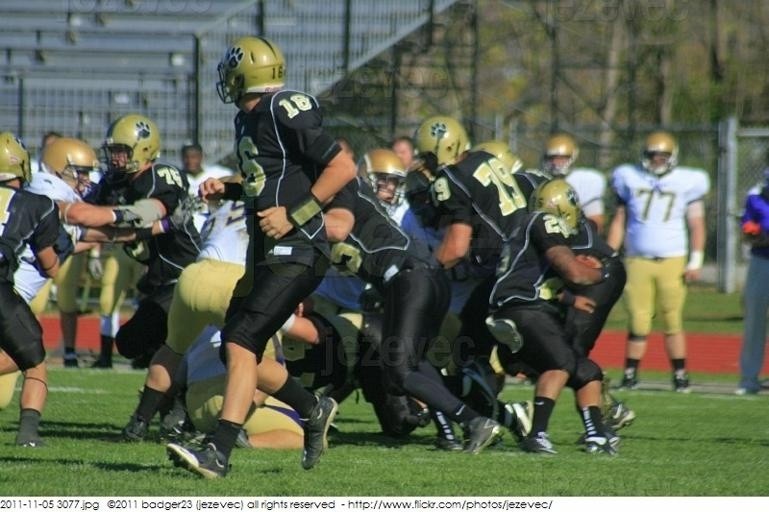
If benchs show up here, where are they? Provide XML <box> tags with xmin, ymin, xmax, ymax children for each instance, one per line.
<box><xmin>0</xmin><ymin>0</ymin><xmax>457</xmax><ymax>174</ymax></box>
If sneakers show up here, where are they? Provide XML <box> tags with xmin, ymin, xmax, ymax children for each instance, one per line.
<box><xmin>506</xmin><ymin>397</ymin><xmax>558</xmax><ymax>456</ymax></box>
<box><xmin>671</xmin><ymin>368</ymin><xmax>692</xmax><ymax>394</ymax></box>
<box><xmin>302</xmin><ymin>393</ymin><xmax>340</xmax><ymax>472</ymax></box>
<box><xmin>122</xmin><ymin>412</ymin><xmax>150</xmax><ymax>442</ymax></box>
<box><xmin>167</xmin><ymin>441</ymin><xmax>228</xmax><ymax>479</ymax></box>
<box><xmin>576</xmin><ymin>400</ymin><xmax>637</xmax><ymax>459</ymax></box>
<box><xmin>618</xmin><ymin>367</ymin><xmax>639</xmax><ymax>392</ymax></box>
<box><xmin>12</xmin><ymin>439</ymin><xmax>55</xmax><ymax>449</ymax></box>
<box><xmin>87</xmin><ymin>359</ymin><xmax>114</xmax><ymax>368</ymax></box>
<box><xmin>459</xmin><ymin>417</ymin><xmax>503</xmax><ymax>457</ymax></box>
<box><xmin>62</xmin><ymin>351</ymin><xmax>81</xmax><ymax>370</ymax></box>
<box><xmin>433</xmin><ymin>435</ymin><xmax>463</xmax><ymax>451</ymax></box>
<box><xmin>161</xmin><ymin>417</ymin><xmax>253</xmax><ymax>448</ymax></box>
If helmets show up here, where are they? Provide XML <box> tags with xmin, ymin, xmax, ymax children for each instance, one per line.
<box><xmin>0</xmin><ymin>129</ymin><xmax>33</xmax><ymax>186</ymax></box>
<box><xmin>103</xmin><ymin>114</ymin><xmax>162</xmax><ymax>176</ymax></box>
<box><xmin>213</xmin><ymin>35</ymin><xmax>288</xmax><ymax>105</ymax></box>
<box><xmin>357</xmin><ymin>149</ymin><xmax>408</xmax><ymax>216</ymax></box>
<box><xmin>639</xmin><ymin>131</ymin><xmax>681</xmax><ymax>175</ymax></box>
<box><xmin>38</xmin><ymin>137</ymin><xmax>102</xmax><ymax>194</ymax></box>
<box><xmin>527</xmin><ymin>176</ymin><xmax>584</xmax><ymax>231</ymax></box>
<box><xmin>474</xmin><ymin>140</ymin><xmax>527</xmax><ymax>175</ymax></box>
<box><xmin>541</xmin><ymin>130</ymin><xmax>579</xmax><ymax>176</ymax></box>
<box><xmin>411</xmin><ymin>115</ymin><xmax>471</xmax><ymax>177</ymax></box>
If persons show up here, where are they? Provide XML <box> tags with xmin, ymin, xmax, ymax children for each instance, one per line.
<box><xmin>735</xmin><ymin>149</ymin><xmax>769</xmax><ymax>396</ymax></box>
<box><xmin>601</xmin><ymin>131</ymin><xmax>711</xmax><ymax>395</ymax></box>
<box><xmin>0</xmin><ymin>36</ymin><xmax>637</xmax><ymax>480</ymax></box>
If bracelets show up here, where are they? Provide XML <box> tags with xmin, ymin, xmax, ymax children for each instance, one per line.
<box><xmin>687</xmin><ymin>252</ymin><xmax>706</xmax><ymax>269</ymax></box>
